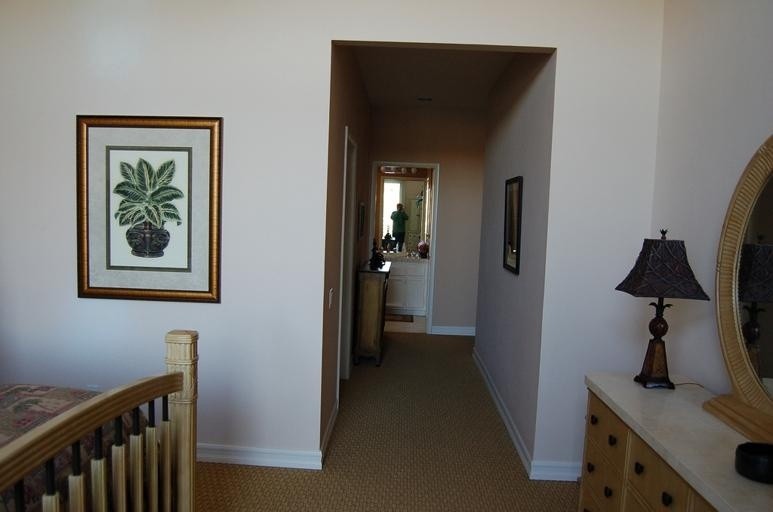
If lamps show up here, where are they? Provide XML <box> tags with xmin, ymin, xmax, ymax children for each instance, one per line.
<box><xmin>612</xmin><ymin>228</ymin><xmax>711</xmax><ymax>392</ymax></box>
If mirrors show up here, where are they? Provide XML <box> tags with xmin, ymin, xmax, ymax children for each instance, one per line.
<box><xmin>702</xmin><ymin>133</ymin><xmax>773</xmax><ymax>444</ymax></box>
<box><xmin>383</xmin><ymin>179</ymin><xmax>426</xmax><ymax>254</ymax></box>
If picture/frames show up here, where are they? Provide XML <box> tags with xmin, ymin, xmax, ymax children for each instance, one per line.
<box><xmin>503</xmin><ymin>175</ymin><xmax>524</xmax><ymax>276</ymax></box>
<box><xmin>74</xmin><ymin>114</ymin><xmax>223</xmax><ymax>305</ymax></box>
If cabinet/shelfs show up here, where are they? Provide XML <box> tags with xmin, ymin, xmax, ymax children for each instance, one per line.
<box><xmin>576</xmin><ymin>369</ymin><xmax>772</xmax><ymax>512</ymax></box>
<box><xmin>354</xmin><ymin>260</ymin><xmax>392</xmax><ymax>367</ymax></box>
<box><xmin>385</xmin><ymin>261</ymin><xmax>428</xmax><ymax>312</ymax></box>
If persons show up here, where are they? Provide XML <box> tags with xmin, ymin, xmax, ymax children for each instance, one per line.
<box><xmin>390</xmin><ymin>203</ymin><xmax>411</xmax><ymax>251</ymax></box>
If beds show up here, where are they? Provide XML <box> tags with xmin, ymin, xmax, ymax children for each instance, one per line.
<box><xmin>0</xmin><ymin>328</ymin><xmax>200</xmax><ymax>510</ymax></box>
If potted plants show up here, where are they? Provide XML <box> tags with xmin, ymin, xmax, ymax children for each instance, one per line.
<box><xmin>111</xmin><ymin>157</ymin><xmax>183</xmax><ymax>260</ymax></box>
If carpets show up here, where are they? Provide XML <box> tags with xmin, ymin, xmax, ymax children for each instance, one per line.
<box><xmin>385</xmin><ymin>313</ymin><xmax>414</xmax><ymax>322</ymax></box>
